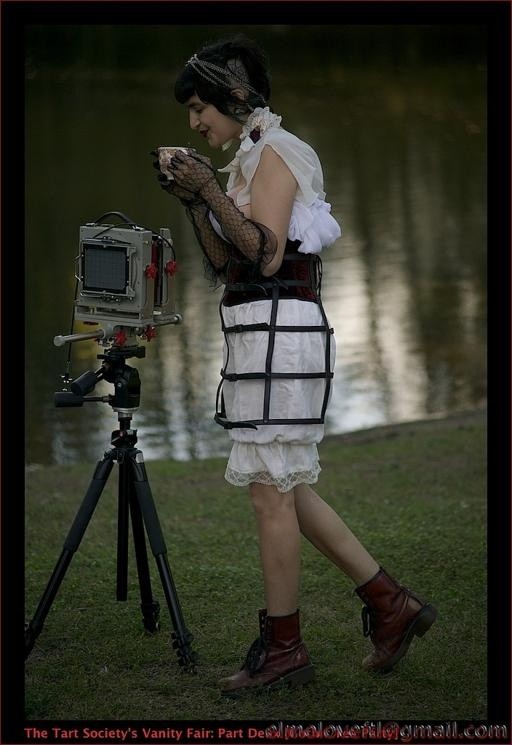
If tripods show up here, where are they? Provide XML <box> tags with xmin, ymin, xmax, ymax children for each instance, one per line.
<box><xmin>25</xmin><ymin>346</ymin><xmax>197</xmax><ymax>672</ymax></box>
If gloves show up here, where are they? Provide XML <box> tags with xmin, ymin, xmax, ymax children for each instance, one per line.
<box><xmin>149</xmin><ymin>147</ymin><xmax>278</xmax><ymax>292</ymax></box>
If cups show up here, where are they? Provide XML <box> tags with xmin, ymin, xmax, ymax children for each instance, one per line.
<box><xmin>157</xmin><ymin>145</ymin><xmax>196</xmax><ymax>181</ymax></box>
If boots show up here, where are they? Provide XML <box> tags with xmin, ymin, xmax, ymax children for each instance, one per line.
<box><xmin>218</xmin><ymin>608</ymin><xmax>315</xmax><ymax>696</ymax></box>
<box><xmin>353</xmin><ymin>566</ymin><xmax>438</xmax><ymax>674</ymax></box>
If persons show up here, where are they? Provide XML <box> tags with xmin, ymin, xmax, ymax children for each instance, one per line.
<box><xmin>147</xmin><ymin>35</ymin><xmax>438</xmax><ymax>700</ymax></box>
